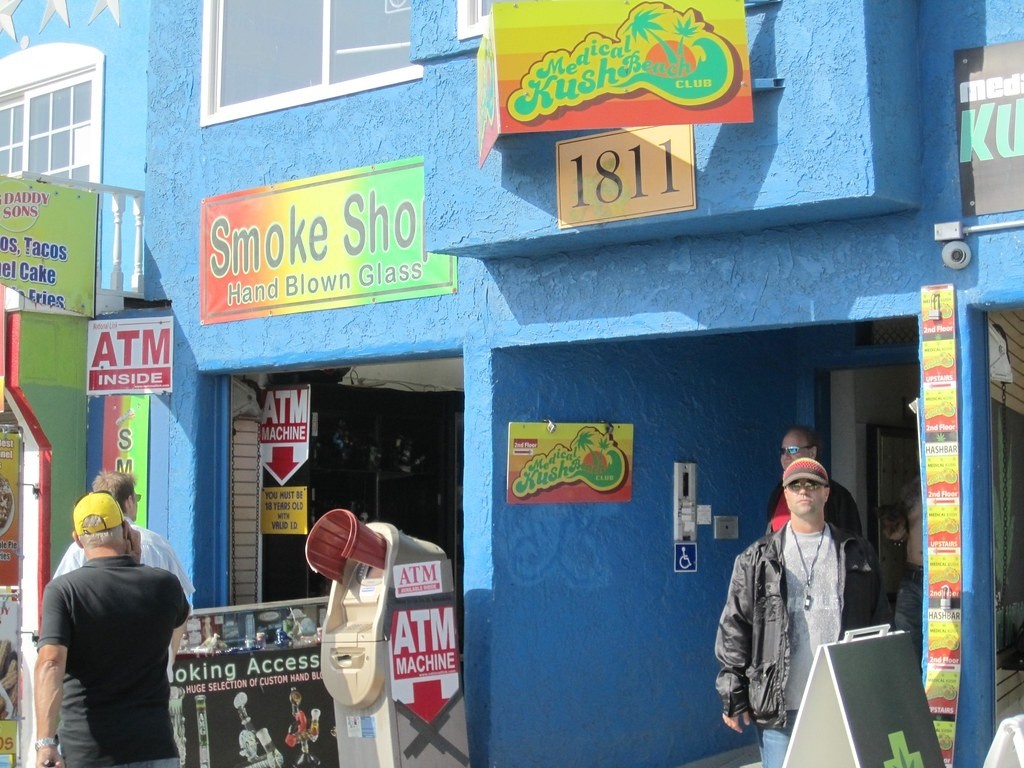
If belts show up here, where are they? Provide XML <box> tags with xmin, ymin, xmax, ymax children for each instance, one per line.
<box><xmin>903</xmin><ymin>568</ymin><xmax>922</xmax><ymax>584</ymax></box>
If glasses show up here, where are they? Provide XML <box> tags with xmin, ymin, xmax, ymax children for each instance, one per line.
<box><xmin>780</xmin><ymin>445</ymin><xmax>815</xmax><ymax>456</ymax></box>
<box><xmin>127</xmin><ymin>494</ymin><xmax>142</xmax><ymax>501</ymax></box>
<box><xmin>74</xmin><ymin>489</ymin><xmax>126</xmax><ymax>527</ymax></box>
<box><xmin>788</xmin><ymin>480</ymin><xmax>821</xmax><ymax>491</ymax></box>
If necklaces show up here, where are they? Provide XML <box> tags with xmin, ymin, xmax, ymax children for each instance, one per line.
<box><xmin>791</xmin><ymin>525</ymin><xmax>826</xmax><ymax>609</ymax></box>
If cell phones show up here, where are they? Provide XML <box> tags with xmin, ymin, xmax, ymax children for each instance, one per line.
<box><xmin>127</xmin><ymin>527</ymin><xmax>133</xmax><ymax>550</ymax></box>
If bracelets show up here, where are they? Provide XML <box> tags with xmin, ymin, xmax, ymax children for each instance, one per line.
<box><xmin>35</xmin><ymin>738</ymin><xmax>57</xmax><ymax>751</ymax></box>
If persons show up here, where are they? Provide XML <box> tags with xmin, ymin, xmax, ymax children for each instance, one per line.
<box><xmin>765</xmin><ymin>424</ymin><xmax>863</xmax><ymax>535</ymax></box>
<box><xmin>52</xmin><ymin>470</ymin><xmax>195</xmax><ymax>685</ymax></box>
<box><xmin>881</xmin><ymin>474</ymin><xmax>923</xmax><ymax>673</ymax></box>
<box><xmin>35</xmin><ymin>490</ymin><xmax>190</xmax><ymax>768</ymax></box>
<box><xmin>715</xmin><ymin>457</ymin><xmax>897</xmax><ymax>768</ymax></box>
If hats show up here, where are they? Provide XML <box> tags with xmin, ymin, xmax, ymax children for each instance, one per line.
<box><xmin>782</xmin><ymin>457</ymin><xmax>829</xmax><ymax>488</ymax></box>
<box><xmin>73</xmin><ymin>492</ymin><xmax>124</xmax><ymax>535</ymax></box>
